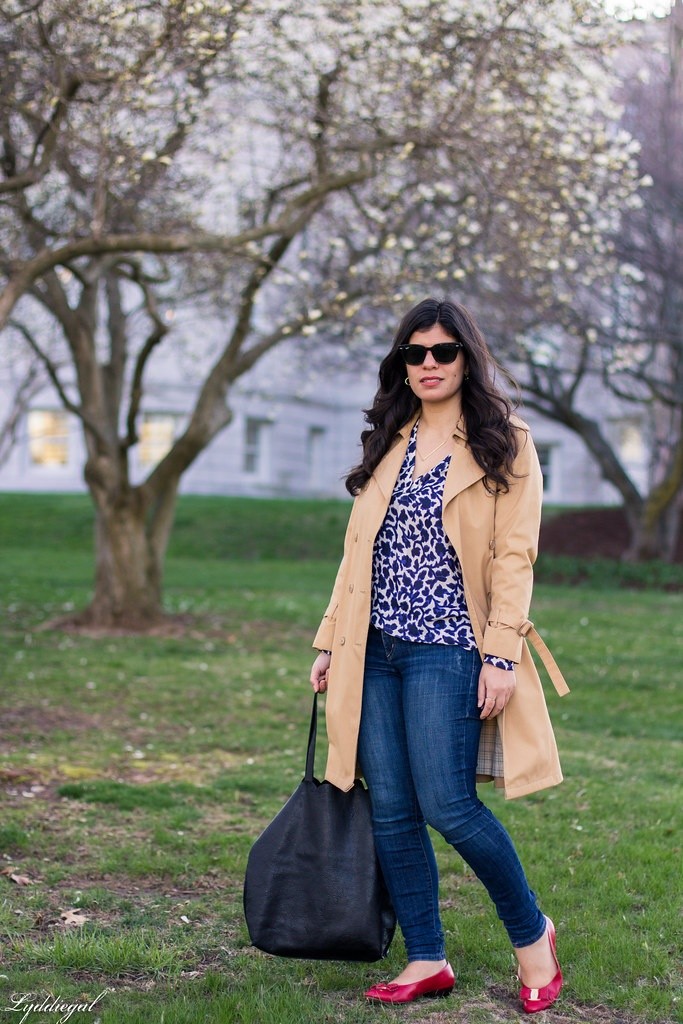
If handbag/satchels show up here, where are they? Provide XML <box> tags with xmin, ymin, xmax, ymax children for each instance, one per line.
<box><xmin>242</xmin><ymin>688</ymin><xmax>402</xmax><ymax>963</ymax></box>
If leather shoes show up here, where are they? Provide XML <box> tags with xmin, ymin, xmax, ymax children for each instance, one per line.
<box><xmin>364</xmin><ymin>962</ymin><xmax>454</xmax><ymax>1003</ymax></box>
<box><xmin>517</xmin><ymin>916</ymin><xmax>563</xmax><ymax>1013</ymax></box>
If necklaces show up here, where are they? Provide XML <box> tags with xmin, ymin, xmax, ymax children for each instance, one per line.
<box><xmin>415</xmin><ymin>425</ymin><xmax>458</xmax><ymax>461</ymax></box>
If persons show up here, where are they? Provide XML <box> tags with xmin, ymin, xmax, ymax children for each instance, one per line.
<box><xmin>310</xmin><ymin>297</ymin><xmax>567</xmax><ymax>1015</ymax></box>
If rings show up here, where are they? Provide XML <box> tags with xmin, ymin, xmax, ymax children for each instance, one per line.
<box><xmin>485</xmin><ymin>696</ymin><xmax>496</xmax><ymax>701</ymax></box>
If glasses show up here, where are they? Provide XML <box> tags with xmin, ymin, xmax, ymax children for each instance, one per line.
<box><xmin>398</xmin><ymin>343</ymin><xmax>464</xmax><ymax>365</ymax></box>
